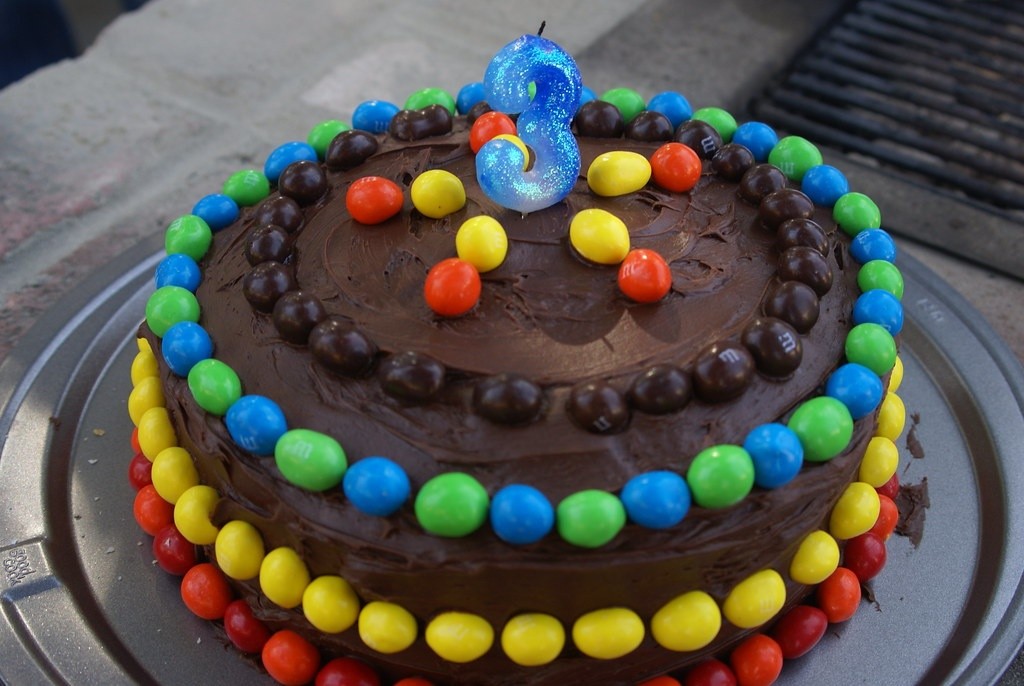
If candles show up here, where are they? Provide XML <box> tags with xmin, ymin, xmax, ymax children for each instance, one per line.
<box><xmin>475</xmin><ymin>20</ymin><xmax>584</xmax><ymax>214</ymax></box>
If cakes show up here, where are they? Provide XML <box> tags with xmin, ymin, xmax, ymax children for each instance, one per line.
<box><xmin>126</xmin><ymin>81</ymin><xmax>906</xmax><ymax>686</ymax></box>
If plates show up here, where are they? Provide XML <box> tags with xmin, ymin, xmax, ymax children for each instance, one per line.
<box><xmin>1</xmin><ymin>224</ymin><xmax>1024</xmax><ymax>686</ymax></box>
